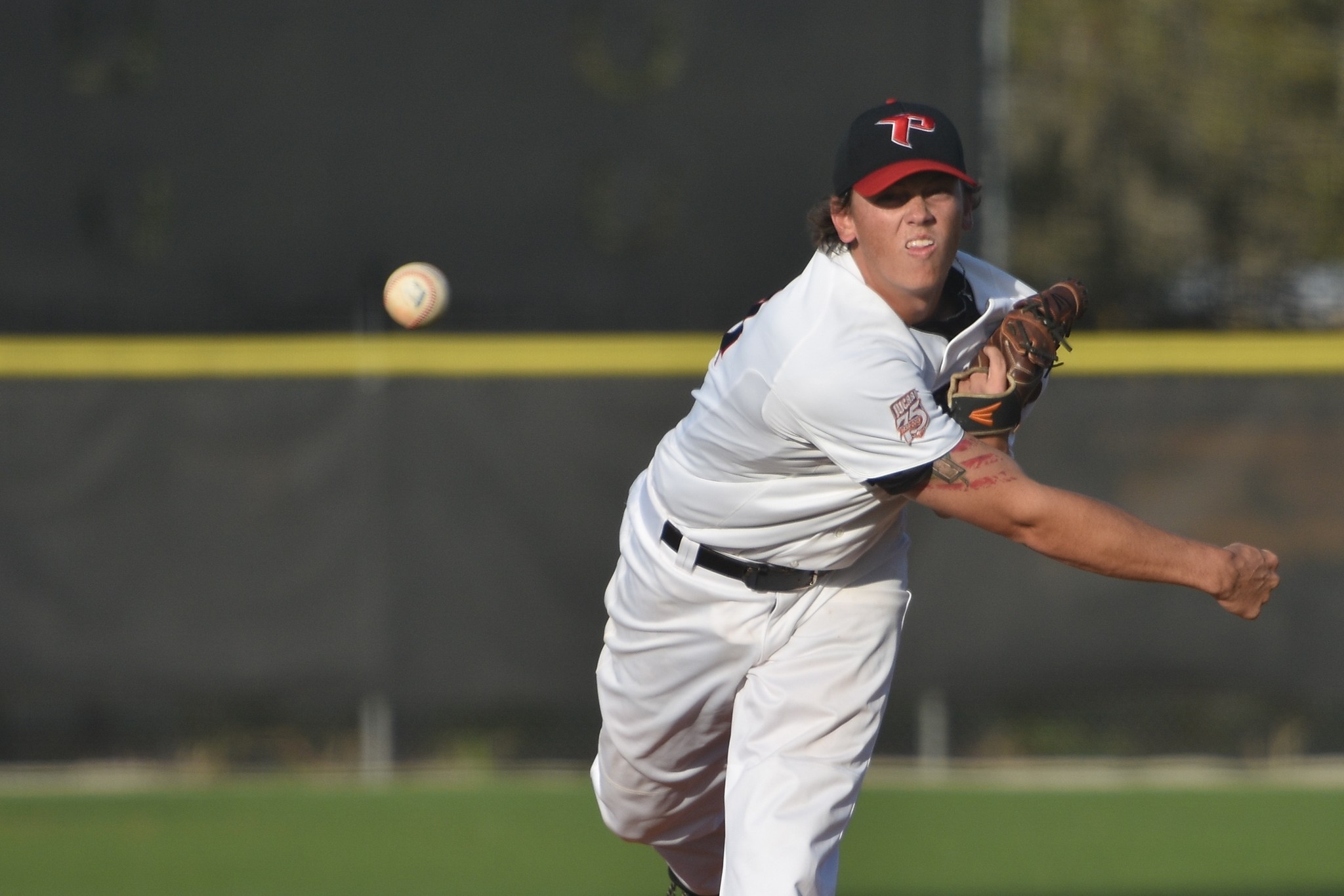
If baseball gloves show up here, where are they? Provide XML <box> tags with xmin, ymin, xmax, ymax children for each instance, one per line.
<box><xmin>947</xmin><ymin>277</ymin><xmax>1088</xmax><ymax>436</ymax></box>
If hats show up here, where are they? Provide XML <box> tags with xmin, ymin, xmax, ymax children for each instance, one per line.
<box><xmin>831</xmin><ymin>99</ymin><xmax>979</xmax><ymax>200</ymax></box>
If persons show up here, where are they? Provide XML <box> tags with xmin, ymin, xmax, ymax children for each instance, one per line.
<box><xmin>587</xmin><ymin>96</ymin><xmax>1281</xmax><ymax>895</ymax></box>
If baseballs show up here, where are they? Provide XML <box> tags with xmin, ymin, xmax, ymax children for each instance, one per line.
<box><xmin>383</xmin><ymin>261</ymin><xmax>452</xmax><ymax>329</ymax></box>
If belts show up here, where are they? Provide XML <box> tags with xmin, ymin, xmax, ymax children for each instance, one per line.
<box><xmin>661</xmin><ymin>521</ymin><xmax>827</xmax><ymax>592</ymax></box>
